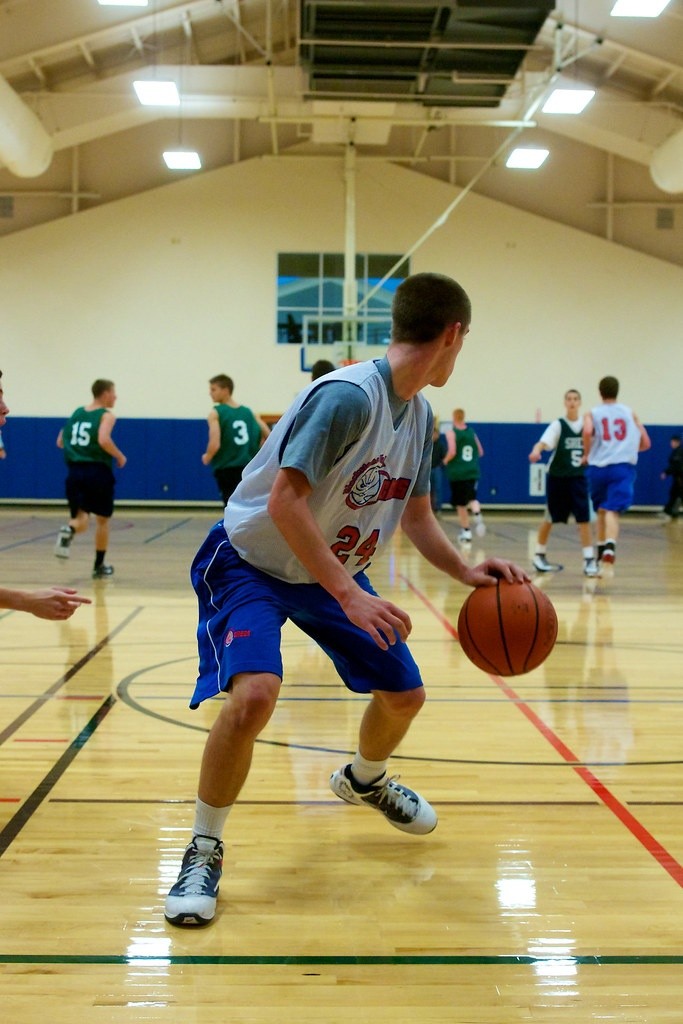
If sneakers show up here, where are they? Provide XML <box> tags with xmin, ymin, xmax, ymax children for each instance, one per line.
<box><xmin>327</xmin><ymin>759</ymin><xmax>438</xmax><ymax>835</ymax></box>
<box><xmin>163</xmin><ymin>833</ymin><xmax>224</xmax><ymax>926</ymax></box>
<box><xmin>532</xmin><ymin>553</ymin><xmax>555</xmax><ymax>571</ymax></box>
<box><xmin>582</xmin><ymin>558</ymin><xmax>599</xmax><ymax>578</ymax></box>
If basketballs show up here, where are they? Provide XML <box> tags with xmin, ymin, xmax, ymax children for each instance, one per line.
<box><xmin>457</xmin><ymin>577</ymin><xmax>560</xmax><ymax>678</ymax></box>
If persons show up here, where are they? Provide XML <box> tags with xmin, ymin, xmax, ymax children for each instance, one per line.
<box><xmin>658</xmin><ymin>437</ymin><xmax>683</xmax><ymax>520</ymax></box>
<box><xmin>164</xmin><ymin>272</ymin><xmax>532</xmax><ymax>928</ymax></box>
<box><xmin>530</xmin><ymin>389</ymin><xmax>596</xmax><ymax>573</ymax></box>
<box><xmin>202</xmin><ymin>375</ymin><xmax>271</xmax><ymax>508</ymax></box>
<box><xmin>53</xmin><ymin>378</ymin><xmax>126</xmax><ymax>576</ymax></box>
<box><xmin>430</xmin><ymin>417</ymin><xmax>448</xmax><ymax>513</ymax></box>
<box><xmin>580</xmin><ymin>376</ymin><xmax>650</xmax><ymax>576</ymax></box>
<box><xmin>0</xmin><ymin>372</ymin><xmax>91</xmax><ymax>620</ymax></box>
<box><xmin>443</xmin><ymin>409</ymin><xmax>485</xmax><ymax>542</ymax></box>
<box><xmin>311</xmin><ymin>360</ymin><xmax>335</xmax><ymax>382</ymax></box>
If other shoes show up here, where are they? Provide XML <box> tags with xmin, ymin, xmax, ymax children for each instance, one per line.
<box><xmin>475</xmin><ymin>513</ymin><xmax>487</xmax><ymax>537</ymax></box>
<box><xmin>458</xmin><ymin>532</ymin><xmax>473</xmax><ymax>548</ymax></box>
<box><xmin>597</xmin><ymin>550</ymin><xmax>615</xmax><ymax>578</ymax></box>
<box><xmin>92</xmin><ymin>563</ymin><xmax>113</xmax><ymax>580</ymax></box>
<box><xmin>53</xmin><ymin>524</ymin><xmax>73</xmax><ymax>564</ymax></box>
